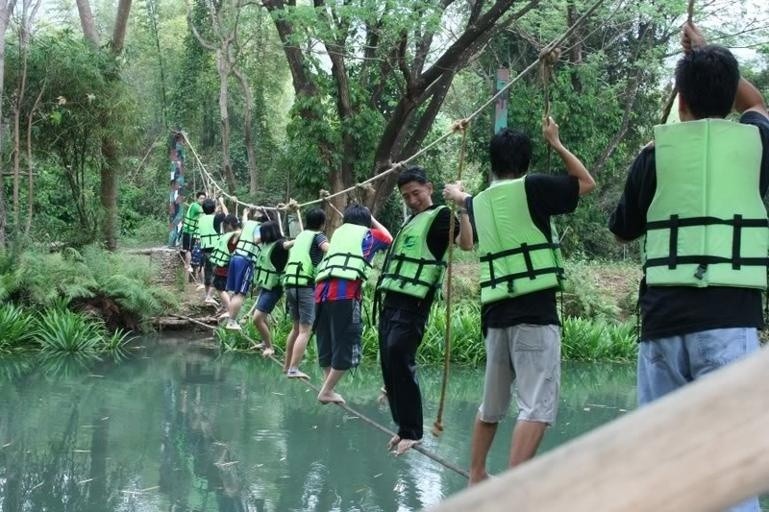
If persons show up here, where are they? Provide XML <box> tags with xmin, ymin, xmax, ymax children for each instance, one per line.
<box><xmin>198</xmin><ymin>197</ymin><xmax>229</xmax><ymax>306</ymax></box>
<box><xmin>442</xmin><ymin>115</ymin><xmax>598</xmax><ymax>491</ymax></box>
<box><xmin>282</xmin><ymin>209</ymin><xmax>331</xmax><ymax>379</ymax></box>
<box><xmin>249</xmin><ymin>221</ymin><xmax>295</xmax><ymax>358</ymax></box>
<box><xmin>314</xmin><ymin>202</ymin><xmax>394</xmax><ymax>406</ymax></box>
<box><xmin>213</xmin><ymin>207</ymin><xmax>250</xmax><ymax>320</ymax></box>
<box><xmin>379</xmin><ymin>168</ymin><xmax>476</xmax><ymax>456</ymax></box>
<box><xmin>192</xmin><ymin>217</ymin><xmax>205</xmax><ymax>293</ymax></box>
<box><xmin>225</xmin><ymin>211</ymin><xmax>274</xmax><ymax>330</ymax></box>
<box><xmin>610</xmin><ymin>24</ymin><xmax>769</xmax><ymax>406</ymax></box>
<box><xmin>182</xmin><ymin>191</ymin><xmax>207</xmax><ymax>272</ymax></box>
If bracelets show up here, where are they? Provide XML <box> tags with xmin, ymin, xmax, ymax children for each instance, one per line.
<box><xmin>456</xmin><ymin>208</ymin><xmax>468</xmax><ymax>215</ymax></box>
